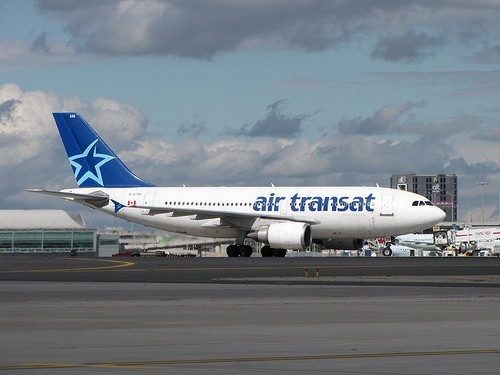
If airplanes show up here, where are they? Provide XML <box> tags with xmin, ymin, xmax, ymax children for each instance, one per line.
<box><xmin>25</xmin><ymin>111</ymin><xmax>447</xmax><ymax>256</ymax></box>
<box><xmin>380</xmin><ymin>224</ymin><xmax>500</xmax><ymax>259</ymax></box>
